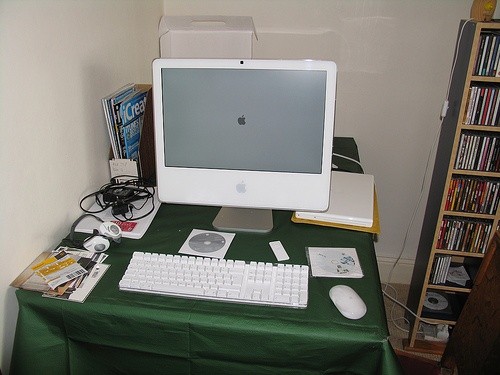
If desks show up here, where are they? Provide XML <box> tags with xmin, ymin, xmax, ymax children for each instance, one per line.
<box><xmin>9</xmin><ymin>137</ymin><xmax>399</xmax><ymax>375</ymax></box>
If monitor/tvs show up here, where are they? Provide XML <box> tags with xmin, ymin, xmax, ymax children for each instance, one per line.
<box><xmin>152</xmin><ymin>58</ymin><xmax>338</xmax><ymax>232</ymax></box>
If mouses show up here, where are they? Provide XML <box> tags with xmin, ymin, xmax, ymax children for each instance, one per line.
<box><xmin>329</xmin><ymin>285</ymin><xmax>367</xmax><ymax>319</ymax></box>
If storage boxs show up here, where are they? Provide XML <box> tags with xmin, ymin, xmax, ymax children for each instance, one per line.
<box><xmin>158</xmin><ymin>13</ymin><xmax>257</xmax><ymax>60</ymax></box>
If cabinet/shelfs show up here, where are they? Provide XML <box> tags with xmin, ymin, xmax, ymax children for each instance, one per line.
<box><xmin>402</xmin><ymin>19</ymin><xmax>500</xmax><ymax>346</ymax></box>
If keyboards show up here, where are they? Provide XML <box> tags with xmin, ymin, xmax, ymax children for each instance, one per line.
<box><xmin>118</xmin><ymin>251</ymin><xmax>309</xmax><ymax>309</ymax></box>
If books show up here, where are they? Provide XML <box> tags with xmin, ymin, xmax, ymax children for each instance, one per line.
<box><xmin>454</xmin><ymin>133</ymin><xmax>500</xmax><ymax>171</ymax></box>
<box><xmin>473</xmin><ymin>35</ymin><xmax>500</xmax><ymax>77</ymax></box>
<box><xmin>102</xmin><ymin>82</ymin><xmax>149</xmax><ymax>164</ymax></box>
<box><xmin>437</xmin><ymin>218</ymin><xmax>492</xmax><ymax>253</ymax></box>
<box><xmin>464</xmin><ymin>87</ymin><xmax>500</xmax><ymax>126</ymax></box>
<box><xmin>444</xmin><ymin>174</ymin><xmax>500</xmax><ymax>215</ymax></box>
<box><xmin>431</xmin><ymin>255</ymin><xmax>470</xmax><ymax>287</ymax></box>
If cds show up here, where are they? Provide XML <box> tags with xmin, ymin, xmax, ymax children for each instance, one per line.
<box><xmin>317</xmin><ymin>251</ymin><xmax>355</xmax><ymax>273</ymax></box>
<box><xmin>423</xmin><ymin>292</ymin><xmax>448</xmax><ymax>310</ymax></box>
<box><xmin>189</xmin><ymin>232</ymin><xmax>226</xmax><ymax>253</ymax></box>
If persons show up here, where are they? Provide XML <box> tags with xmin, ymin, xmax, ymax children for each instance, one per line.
<box><xmin>440</xmin><ymin>231</ymin><xmax>500</xmax><ymax>375</ymax></box>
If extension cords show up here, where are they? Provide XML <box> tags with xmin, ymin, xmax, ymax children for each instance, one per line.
<box><xmin>436</xmin><ymin>325</ymin><xmax>449</xmax><ymax>337</ymax></box>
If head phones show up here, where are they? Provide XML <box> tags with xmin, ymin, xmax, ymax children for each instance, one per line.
<box><xmin>70</xmin><ymin>214</ymin><xmax>122</xmax><ymax>253</ymax></box>
<box><xmin>103</xmin><ymin>188</ymin><xmax>134</xmax><ymax>202</ymax></box>
